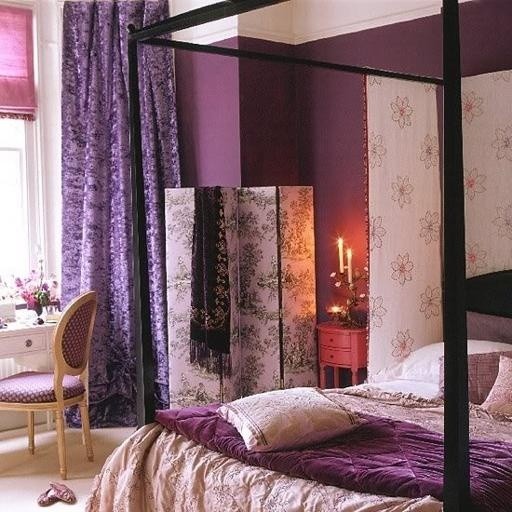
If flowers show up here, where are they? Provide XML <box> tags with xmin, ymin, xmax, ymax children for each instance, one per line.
<box><xmin>1</xmin><ymin>261</ymin><xmax>62</xmax><ymax>324</ymax></box>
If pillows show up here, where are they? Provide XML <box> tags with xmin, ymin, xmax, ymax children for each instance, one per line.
<box><xmin>216</xmin><ymin>385</ymin><xmax>371</xmax><ymax>455</ymax></box>
<box><xmin>437</xmin><ymin>351</ymin><xmax>508</xmax><ymax>404</ymax></box>
<box><xmin>388</xmin><ymin>339</ymin><xmax>512</xmax><ymax>384</ymax></box>
<box><xmin>480</xmin><ymin>356</ymin><xmax>512</xmax><ymax>420</ymax></box>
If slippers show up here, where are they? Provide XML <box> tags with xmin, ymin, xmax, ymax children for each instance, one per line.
<box><xmin>38</xmin><ymin>481</ymin><xmax>75</xmax><ymax>506</ymax></box>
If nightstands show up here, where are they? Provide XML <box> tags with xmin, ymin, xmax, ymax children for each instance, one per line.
<box><xmin>314</xmin><ymin>321</ymin><xmax>369</xmax><ymax>388</ymax></box>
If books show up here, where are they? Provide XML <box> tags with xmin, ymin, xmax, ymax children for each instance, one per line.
<box><xmin>46</xmin><ymin>314</ymin><xmax>60</xmax><ymax>323</ymax></box>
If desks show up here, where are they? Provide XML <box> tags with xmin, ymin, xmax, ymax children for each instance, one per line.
<box><xmin>0</xmin><ymin>317</ymin><xmax>57</xmax><ymax>430</ymax></box>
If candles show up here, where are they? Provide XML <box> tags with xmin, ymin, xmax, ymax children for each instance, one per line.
<box><xmin>346</xmin><ymin>249</ymin><xmax>353</xmax><ymax>285</ymax></box>
<box><xmin>338</xmin><ymin>238</ymin><xmax>344</xmax><ymax>272</ymax></box>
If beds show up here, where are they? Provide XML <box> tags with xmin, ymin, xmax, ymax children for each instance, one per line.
<box><xmin>82</xmin><ymin>0</ymin><xmax>510</xmax><ymax>512</ymax></box>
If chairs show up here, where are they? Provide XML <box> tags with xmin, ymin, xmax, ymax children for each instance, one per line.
<box><xmin>1</xmin><ymin>291</ymin><xmax>99</xmax><ymax>480</ymax></box>
